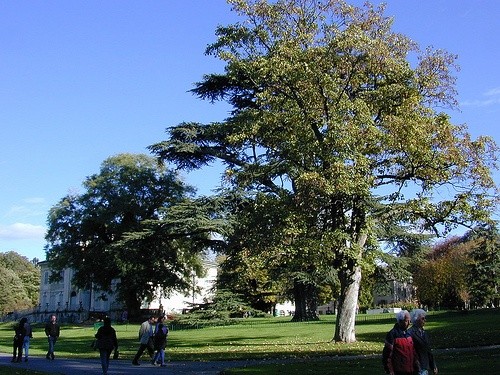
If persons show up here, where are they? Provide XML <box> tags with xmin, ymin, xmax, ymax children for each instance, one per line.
<box><xmin>406</xmin><ymin>309</ymin><xmax>438</xmax><ymax>375</ymax></box>
<box><xmin>11</xmin><ymin>320</ymin><xmax>26</xmax><ymax>362</ymax></box>
<box><xmin>45</xmin><ymin>313</ymin><xmax>60</xmax><ymax>360</ymax></box>
<box><xmin>383</xmin><ymin>310</ymin><xmax>420</xmax><ymax>375</ymax></box>
<box><xmin>95</xmin><ymin>317</ymin><xmax>118</xmax><ymax>375</ymax></box>
<box><xmin>21</xmin><ymin>318</ymin><xmax>33</xmax><ymax>362</ymax></box>
<box><xmin>132</xmin><ymin>316</ymin><xmax>168</xmax><ymax>367</ymax></box>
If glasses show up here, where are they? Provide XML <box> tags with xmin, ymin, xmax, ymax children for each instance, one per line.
<box><xmin>422</xmin><ymin>316</ymin><xmax>427</xmax><ymax>320</ymax></box>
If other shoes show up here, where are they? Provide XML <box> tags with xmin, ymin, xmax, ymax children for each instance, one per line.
<box><xmin>153</xmin><ymin>360</ymin><xmax>157</xmax><ymax>366</ymax></box>
<box><xmin>132</xmin><ymin>360</ymin><xmax>140</xmax><ymax>366</ymax></box>
<box><xmin>42</xmin><ymin>353</ymin><xmax>55</xmax><ymax>362</ymax></box>
<box><xmin>161</xmin><ymin>362</ymin><xmax>168</xmax><ymax>367</ymax></box>
